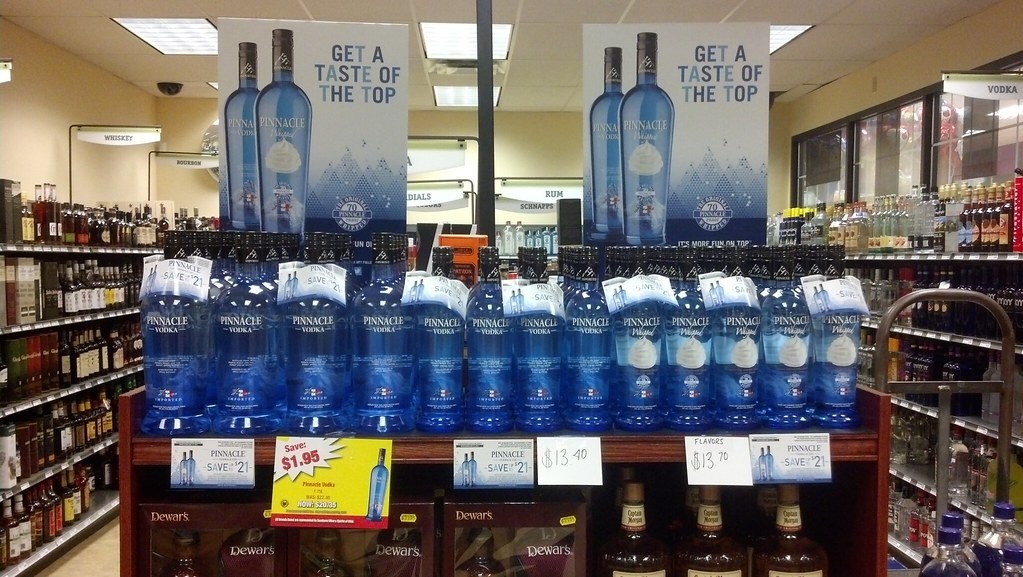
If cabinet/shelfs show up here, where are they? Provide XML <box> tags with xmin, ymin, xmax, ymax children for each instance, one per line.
<box><xmin>118</xmin><ymin>248</ymin><xmax>1022</xmax><ymax>577</ymax></box>
<box><xmin>0</xmin><ymin>242</ymin><xmax>164</xmax><ymax>577</ymax></box>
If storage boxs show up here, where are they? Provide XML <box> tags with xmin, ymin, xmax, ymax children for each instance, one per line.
<box><xmin>0</xmin><ymin>179</ymin><xmax>23</xmax><ymax>244</ymax></box>
<box><xmin>443</xmin><ymin>482</ymin><xmax>588</xmax><ymax>577</ymax></box>
<box><xmin>139</xmin><ymin>486</ymin><xmax>287</xmax><ymax>577</ymax></box>
<box><xmin>0</xmin><ymin>255</ymin><xmax>59</xmax><ymax>327</ymax></box>
<box><xmin>286</xmin><ymin>488</ymin><xmax>435</xmax><ymax>577</ymax></box>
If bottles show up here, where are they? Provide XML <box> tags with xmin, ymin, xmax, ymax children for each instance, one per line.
<box><xmin>224</xmin><ymin>41</ymin><xmax>260</xmax><ymax>230</ymax></box>
<box><xmin>759</xmin><ymin>448</ymin><xmax>767</xmax><ymax>480</ymax></box>
<box><xmin>253</xmin><ymin>29</ymin><xmax>312</xmax><ymax>232</ymax></box>
<box><xmin>0</xmin><ymin>182</ymin><xmax>1023</xmax><ymax>577</ymax></box>
<box><xmin>180</xmin><ymin>452</ymin><xmax>188</xmax><ymax>484</ymax></box>
<box><xmin>590</xmin><ymin>47</ymin><xmax>626</xmax><ymax>244</ymax></box>
<box><xmin>462</xmin><ymin>453</ymin><xmax>469</xmax><ymax>486</ymax></box>
<box><xmin>365</xmin><ymin>448</ymin><xmax>388</xmax><ymax>521</ymax></box>
<box><xmin>186</xmin><ymin>450</ymin><xmax>196</xmax><ymax>484</ymax></box>
<box><xmin>468</xmin><ymin>452</ymin><xmax>477</xmax><ymax>486</ymax></box>
<box><xmin>617</xmin><ymin>33</ymin><xmax>675</xmax><ymax>244</ymax></box>
<box><xmin>765</xmin><ymin>446</ymin><xmax>773</xmax><ymax>480</ymax></box>
